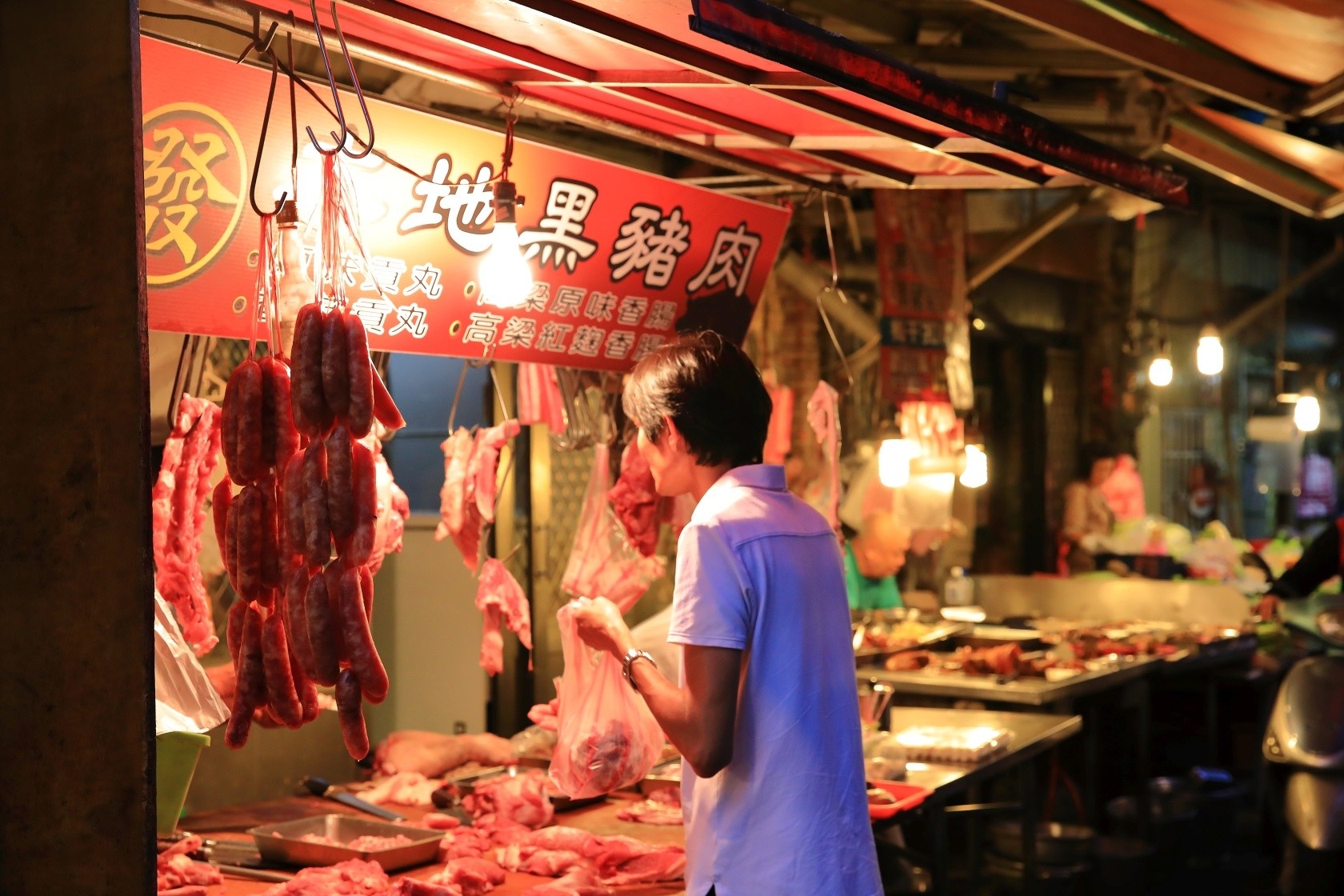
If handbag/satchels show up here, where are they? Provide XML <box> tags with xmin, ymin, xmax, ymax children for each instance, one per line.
<box><xmin>546</xmin><ymin>599</ymin><xmax>664</xmax><ymax>798</ymax></box>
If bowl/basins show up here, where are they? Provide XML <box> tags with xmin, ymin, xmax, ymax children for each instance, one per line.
<box><xmin>245</xmin><ymin>814</ymin><xmax>449</xmax><ymax>868</ymax></box>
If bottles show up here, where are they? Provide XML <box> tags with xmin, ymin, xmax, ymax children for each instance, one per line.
<box><xmin>945</xmin><ymin>565</ymin><xmax>975</xmax><ymax>605</ymax></box>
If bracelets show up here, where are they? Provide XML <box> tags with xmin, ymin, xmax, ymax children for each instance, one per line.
<box><xmin>620</xmin><ymin>649</ymin><xmax>657</xmax><ymax>694</ymax></box>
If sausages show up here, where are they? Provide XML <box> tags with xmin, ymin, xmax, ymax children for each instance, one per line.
<box><xmin>222</xmin><ymin>300</ymin><xmax>417</xmax><ymax>764</ymax></box>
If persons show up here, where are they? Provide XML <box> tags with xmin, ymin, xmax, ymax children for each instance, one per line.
<box><xmin>569</xmin><ymin>330</ymin><xmax>884</xmax><ymax>896</ymax></box>
<box><xmin>1248</xmin><ymin>511</ymin><xmax>1344</xmax><ymax>622</ymax></box>
<box><xmin>1057</xmin><ymin>441</ymin><xmax>1127</xmax><ymax>576</ymax></box>
<box><xmin>151</xmin><ymin>414</ymin><xmax>280</xmax><ymax>730</ymax></box>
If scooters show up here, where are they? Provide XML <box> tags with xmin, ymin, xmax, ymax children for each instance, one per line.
<box><xmin>1238</xmin><ymin>550</ymin><xmax>1344</xmax><ymax>896</ymax></box>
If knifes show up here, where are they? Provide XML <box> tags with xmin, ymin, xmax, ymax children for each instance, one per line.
<box><xmin>299</xmin><ymin>774</ymin><xmax>408</xmax><ymax>822</ymax></box>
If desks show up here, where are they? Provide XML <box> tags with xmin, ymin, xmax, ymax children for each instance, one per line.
<box><xmin>154</xmin><ymin>703</ymin><xmax>1086</xmax><ymax>896</ymax></box>
<box><xmin>852</xmin><ymin>618</ymin><xmax>1261</xmax><ymax>896</ymax></box>
